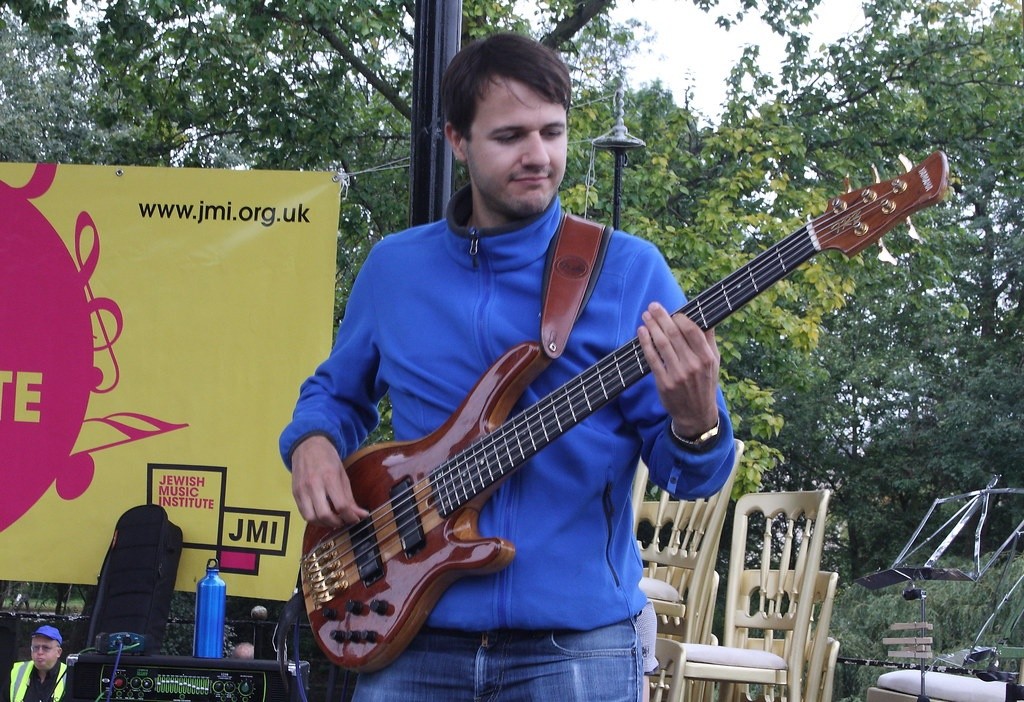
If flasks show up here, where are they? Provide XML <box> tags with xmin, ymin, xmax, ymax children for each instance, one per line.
<box><xmin>193</xmin><ymin>558</ymin><xmax>226</xmax><ymax>659</ymax></box>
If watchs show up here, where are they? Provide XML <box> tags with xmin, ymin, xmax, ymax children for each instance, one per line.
<box><xmin>669</xmin><ymin>412</ymin><xmax>723</xmax><ymax>449</ymax></box>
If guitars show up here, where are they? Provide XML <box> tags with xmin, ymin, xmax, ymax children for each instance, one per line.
<box><xmin>299</xmin><ymin>149</ymin><xmax>959</xmax><ymax>673</ymax></box>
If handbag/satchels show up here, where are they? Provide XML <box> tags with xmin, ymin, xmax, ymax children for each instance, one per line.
<box><xmin>83</xmin><ymin>503</ymin><xmax>182</xmax><ymax>655</ymax></box>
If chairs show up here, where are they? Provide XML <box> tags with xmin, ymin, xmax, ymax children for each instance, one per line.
<box><xmin>630</xmin><ymin>439</ymin><xmax>841</xmax><ymax>702</ymax></box>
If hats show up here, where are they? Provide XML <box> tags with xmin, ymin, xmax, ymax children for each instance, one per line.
<box><xmin>32</xmin><ymin>625</ymin><xmax>63</xmax><ymax>646</ymax></box>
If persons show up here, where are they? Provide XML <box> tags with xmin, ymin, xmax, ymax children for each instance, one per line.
<box><xmin>230</xmin><ymin>642</ymin><xmax>254</xmax><ymax>659</ymax></box>
<box><xmin>3</xmin><ymin>625</ymin><xmax>70</xmax><ymax>702</ymax></box>
<box><xmin>277</xmin><ymin>34</ymin><xmax>738</xmax><ymax>702</ymax></box>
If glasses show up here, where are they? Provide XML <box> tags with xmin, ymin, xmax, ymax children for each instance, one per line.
<box><xmin>31</xmin><ymin>645</ymin><xmax>52</xmax><ymax>653</ymax></box>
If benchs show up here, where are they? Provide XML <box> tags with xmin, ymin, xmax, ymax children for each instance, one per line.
<box><xmin>868</xmin><ymin>668</ymin><xmax>1007</xmax><ymax>702</ymax></box>
